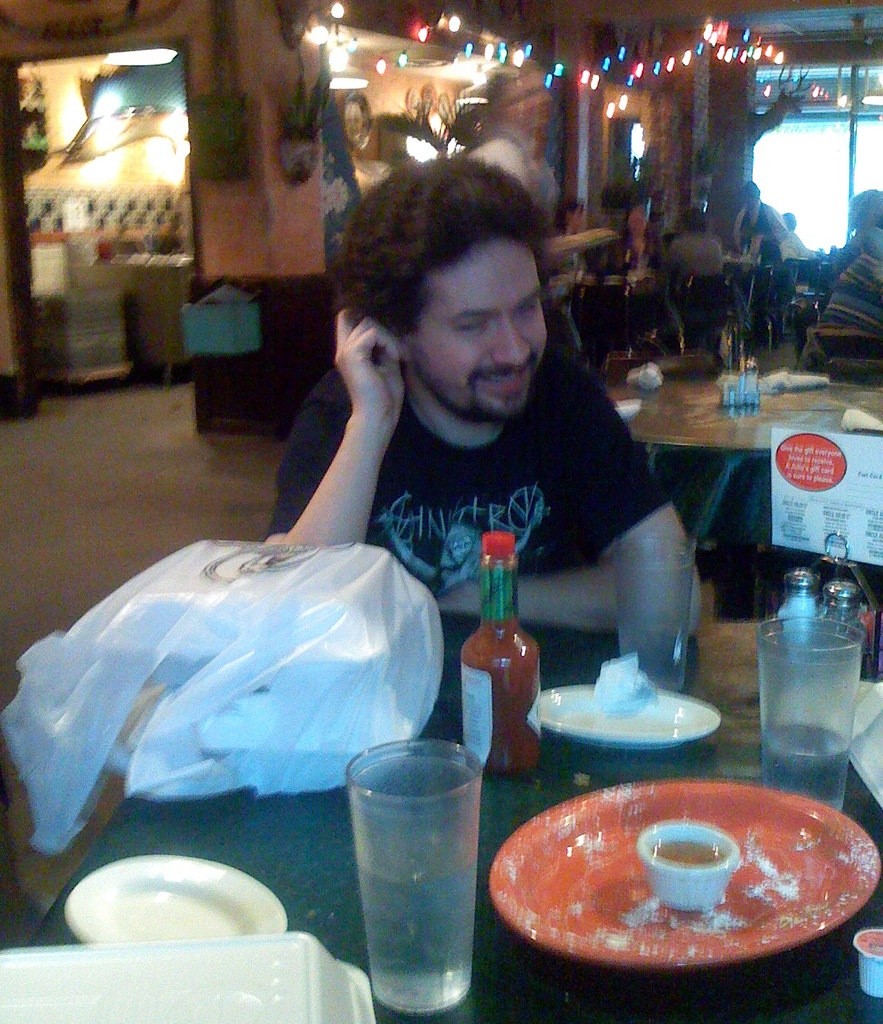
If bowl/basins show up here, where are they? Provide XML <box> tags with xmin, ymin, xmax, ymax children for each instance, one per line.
<box><xmin>636</xmin><ymin>821</ymin><xmax>740</xmax><ymax>912</ymax></box>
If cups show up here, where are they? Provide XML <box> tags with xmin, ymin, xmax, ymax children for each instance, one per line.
<box><xmin>757</xmin><ymin>617</ymin><xmax>865</xmax><ymax>812</ymax></box>
<box><xmin>347</xmin><ymin>740</ymin><xmax>483</xmax><ymax>1016</ymax></box>
<box><xmin>608</xmin><ymin>541</ymin><xmax>696</xmax><ymax>691</ymax></box>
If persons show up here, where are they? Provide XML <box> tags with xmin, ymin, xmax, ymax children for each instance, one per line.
<box><xmin>553</xmin><ymin>181</ymin><xmax>815</xmax><ymax>370</ymax></box>
<box><xmin>263</xmin><ymin>156</ymin><xmax>705</xmax><ymax>637</ymax></box>
<box><xmin>816</xmin><ymin>190</ymin><xmax>883</xmax><ymax>361</ymax></box>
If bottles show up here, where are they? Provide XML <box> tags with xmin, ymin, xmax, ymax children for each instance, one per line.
<box><xmin>461</xmin><ymin>531</ymin><xmax>540</xmax><ymax>770</ymax></box>
<box><xmin>775</xmin><ymin>570</ymin><xmax>819</xmax><ymax>618</ymax></box>
<box><xmin>819</xmin><ymin>580</ymin><xmax>867</xmax><ymax>654</ymax></box>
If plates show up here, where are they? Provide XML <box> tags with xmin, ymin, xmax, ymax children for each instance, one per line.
<box><xmin>490</xmin><ymin>780</ymin><xmax>878</xmax><ymax>971</ymax></box>
<box><xmin>540</xmin><ymin>687</ymin><xmax>720</xmax><ymax>748</ymax></box>
<box><xmin>63</xmin><ymin>855</ymin><xmax>286</xmax><ymax>944</ymax></box>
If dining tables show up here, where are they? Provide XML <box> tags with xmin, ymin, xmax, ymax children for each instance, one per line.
<box><xmin>608</xmin><ymin>383</ymin><xmax>883</xmax><ymax>540</ymax></box>
<box><xmin>26</xmin><ymin>623</ymin><xmax>883</xmax><ymax>1024</ymax></box>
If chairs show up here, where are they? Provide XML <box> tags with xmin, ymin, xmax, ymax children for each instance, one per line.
<box><xmin>722</xmin><ymin>259</ymin><xmax>798</xmax><ymax>351</ymax></box>
